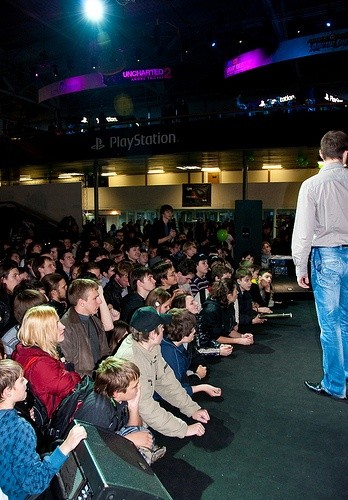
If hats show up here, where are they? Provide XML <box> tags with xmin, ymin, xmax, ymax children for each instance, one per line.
<box><xmin>191</xmin><ymin>252</ymin><xmax>208</xmax><ymax>262</ymax></box>
<box><xmin>130</xmin><ymin>306</ymin><xmax>174</xmax><ymax>332</ymax></box>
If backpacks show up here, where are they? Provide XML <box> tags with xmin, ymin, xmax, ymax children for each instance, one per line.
<box><xmin>14</xmin><ymin>383</ymin><xmax>48</xmax><ymax>452</ymax></box>
<box><xmin>43</xmin><ymin>374</ymin><xmax>96</xmax><ymax>452</ymax></box>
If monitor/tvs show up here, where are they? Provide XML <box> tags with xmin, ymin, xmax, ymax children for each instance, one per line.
<box><xmin>182</xmin><ymin>184</ymin><xmax>212</xmax><ymax>207</ymax></box>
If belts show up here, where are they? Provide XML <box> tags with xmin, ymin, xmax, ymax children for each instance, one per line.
<box><xmin>311</xmin><ymin>244</ymin><xmax>348</xmax><ymax>248</ymax></box>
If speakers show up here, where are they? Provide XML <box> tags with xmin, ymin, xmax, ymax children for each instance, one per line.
<box><xmin>234</xmin><ymin>200</ymin><xmax>263</xmax><ymax>267</ymax></box>
<box><xmin>52</xmin><ymin>419</ymin><xmax>173</xmax><ymax>500</ymax></box>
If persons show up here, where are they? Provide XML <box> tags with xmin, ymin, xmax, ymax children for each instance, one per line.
<box><xmin>291</xmin><ymin>130</ymin><xmax>348</xmax><ymax>400</ymax></box>
<box><xmin>0</xmin><ymin>204</ymin><xmax>297</xmax><ymax>500</ymax></box>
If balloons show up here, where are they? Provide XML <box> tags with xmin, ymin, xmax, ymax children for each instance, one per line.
<box><xmin>217</xmin><ymin>228</ymin><xmax>228</xmax><ymax>241</ymax></box>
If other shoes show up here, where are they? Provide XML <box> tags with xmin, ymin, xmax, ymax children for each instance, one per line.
<box><xmin>305</xmin><ymin>378</ymin><xmax>346</xmax><ymax>400</ymax></box>
